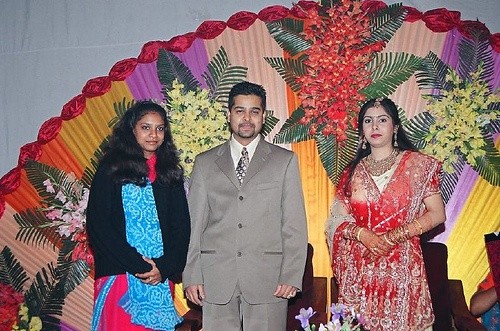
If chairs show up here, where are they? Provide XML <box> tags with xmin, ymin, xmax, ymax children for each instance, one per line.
<box><xmin>175</xmin><ymin>243</ymin><xmax>327</xmax><ymax>331</ymax></box>
<box><xmin>330</xmin><ymin>242</ymin><xmax>486</xmax><ymax>331</ymax></box>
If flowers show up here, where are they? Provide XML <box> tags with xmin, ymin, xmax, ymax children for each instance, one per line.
<box><xmin>12</xmin><ymin>134</ymin><xmax>111</xmax><ymax>299</ymax></box>
<box><xmin>404</xmin><ymin>17</ymin><xmax>500</xmax><ymax>207</ymax></box>
<box><xmin>263</xmin><ymin>0</ymin><xmax>425</xmax><ymax>187</ymax></box>
<box><xmin>295</xmin><ymin>303</ymin><xmax>364</xmax><ymax>331</ymax></box>
<box><xmin>107</xmin><ymin>46</ymin><xmax>279</xmax><ymax>189</ymax></box>
<box><xmin>0</xmin><ymin>245</ymin><xmax>72</xmax><ymax>331</ymax></box>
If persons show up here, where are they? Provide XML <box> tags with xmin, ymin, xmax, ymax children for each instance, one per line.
<box><xmin>183</xmin><ymin>81</ymin><xmax>309</xmax><ymax>331</ymax></box>
<box><xmin>89</xmin><ymin>101</ymin><xmax>191</xmax><ymax>331</ymax></box>
<box><xmin>471</xmin><ymin>270</ymin><xmax>498</xmax><ymax>318</ymax></box>
<box><xmin>325</xmin><ymin>98</ymin><xmax>447</xmax><ymax>330</ymax></box>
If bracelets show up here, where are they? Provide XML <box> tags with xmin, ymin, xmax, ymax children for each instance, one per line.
<box><xmin>357</xmin><ymin>227</ymin><xmax>365</xmax><ymax>241</ymax></box>
<box><xmin>384</xmin><ymin>218</ymin><xmax>424</xmax><ymax>245</ymax></box>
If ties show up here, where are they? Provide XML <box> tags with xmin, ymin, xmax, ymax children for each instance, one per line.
<box><xmin>235</xmin><ymin>146</ymin><xmax>248</xmax><ymax>186</ymax></box>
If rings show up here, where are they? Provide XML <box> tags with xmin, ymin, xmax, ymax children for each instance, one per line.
<box><xmin>288</xmin><ymin>293</ymin><xmax>293</xmax><ymax>296</ymax></box>
<box><xmin>374</xmin><ymin>247</ymin><xmax>376</xmax><ymax>251</ymax></box>
<box><xmin>149</xmin><ymin>277</ymin><xmax>152</xmax><ymax>282</ymax></box>
